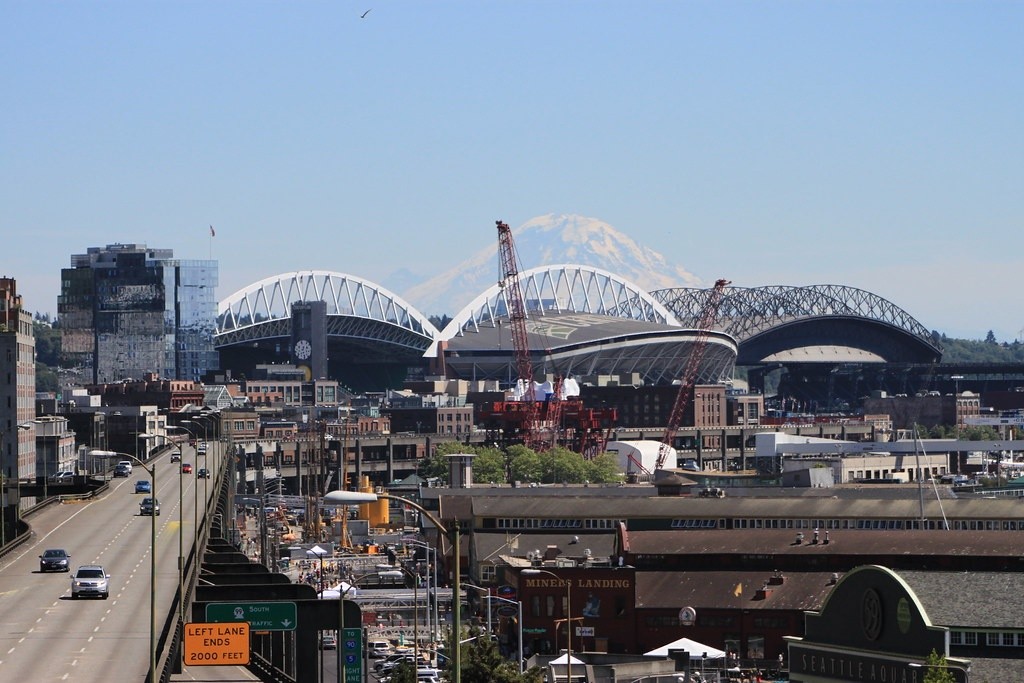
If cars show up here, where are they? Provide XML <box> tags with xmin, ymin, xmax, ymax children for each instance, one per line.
<box><xmin>198</xmin><ymin>469</ymin><xmax>210</xmax><ymax>480</ymax></box>
<box><xmin>133</xmin><ymin>480</ymin><xmax>151</xmax><ymax>493</ymax></box>
<box><xmin>187</xmin><ymin>439</ymin><xmax>197</xmax><ymax>446</ymax></box>
<box><xmin>179</xmin><ymin>463</ymin><xmax>193</xmax><ymax>474</ymax></box>
<box><xmin>197</xmin><ymin>447</ymin><xmax>207</xmax><ymax>456</ymax></box>
<box><xmin>219</xmin><ymin>434</ymin><xmax>228</xmax><ymax>442</ymax></box>
<box><xmin>114</xmin><ymin>465</ymin><xmax>129</xmax><ymax>477</ymax></box>
<box><xmin>319</xmin><ymin>635</ymin><xmax>335</xmax><ymax>649</ymax></box>
<box><xmin>39</xmin><ymin>548</ymin><xmax>71</xmax><ymax>573</ymax></box>
<box><xmin>200</xmin><ymin>442</ymin><xmax>210</xmax><ymax>449</ymax></box>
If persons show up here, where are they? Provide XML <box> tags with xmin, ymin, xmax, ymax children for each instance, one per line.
<box><xmin>296</xmin><ymin>560</ymin><xmax>355</xmax><ymax>593</ymax></box>
<box><xmin>729</xmin><ymin>648</ymin><xmax>784</xmax><ymax>683</ymax></box>
<box><xmin>368</xmin><ymin>613</ymin><xmax>403</xmax><ymax>629</ymax></box>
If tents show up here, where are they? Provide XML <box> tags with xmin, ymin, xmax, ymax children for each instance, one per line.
<box><xmin>643</xmin><ymin>638</ymin><xmax>726</xmax><ymax>671</ymax></box>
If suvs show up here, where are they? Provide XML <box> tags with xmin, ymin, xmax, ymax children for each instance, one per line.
<box><xmin>171</xmin><ymin>451</ymin><xmax>181</xmax><ymax>463</ymax></box>
<box><xmin>139</xmin><ymin>497</ymin><xmax>161</xmax><ymax>516</ymax></box>
<box><xmin>70</xmin><ymin>565</ymin><xmax>111</xmax><ymax>600</ymax></box>
<box><xmin>118</xmin><ymin>461</ymin><xmax>133</xmax><ymax>474</ymax></box>
<box><xmin>362</xmin><ymin>641</ymin><xmax>428</xmax><ymax>678</ymax></box>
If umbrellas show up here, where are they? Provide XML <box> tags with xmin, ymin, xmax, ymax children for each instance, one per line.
<box><xmin>549</xmin><ymin>653</ymin><xmax>584</xmax><ymax>664</ymax></box>
<box><xmin>318</xmin><ymin>582</ymin><xmax>357</xmax><ymax>600</ymax></box>
<box><xmin>307</xmin><ymin>545</ymin><xmax>327</xmax><ymax>554</ymax></box>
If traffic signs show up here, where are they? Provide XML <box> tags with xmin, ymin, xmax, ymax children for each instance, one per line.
<box><xmin>205</xmin><ymin>602</ymin><xmax>298</xmax><ymax>632</ymax></box>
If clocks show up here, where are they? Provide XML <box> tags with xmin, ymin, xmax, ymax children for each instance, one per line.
<box><xmin>294</xmin><ymin>339</ymin><xmax>312</xmax><ymax>360</ymax></box>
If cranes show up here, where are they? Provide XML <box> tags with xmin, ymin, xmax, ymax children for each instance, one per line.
<box><xmin>493</xmin><ymin>220</ymin><xmax>566</xmax><ymax>454</ymax></box>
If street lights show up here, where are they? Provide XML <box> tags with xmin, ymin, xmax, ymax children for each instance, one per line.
<box><xmin>139</xmin><ymin>433</ymin><xmax>183</xmax><ymax>619</ymax></box>
<box><xmin>482</xmin><ymin>568</ymin><xmax>570</xmax><ymax>683</ymax></box>
<box><xmin>322</xmin><ymin>490</ymin><xmax>461</xmax><ymax>683</ymax></box>
<box><xmin>162</xmin><ymin>410</ymin><xmax>222</xmax><ymax>565</ymax></box>
<box><xmin>88</xmin><ymin>449</ymin><xmax>159</xmax><ymax>683</ymax></box>
<box><xmin>340</xmin><ymin>565</ymin><xmax>402</xmax><ymax>683</ymax></box>
<box><xmin>372</xmin><ymin>564</ymin><xmax>418</xmax><ymax>683</ymax></box>
<box><xmin>398</xmin><ymin>537</ymin><xmax>439</xmax><ymax>666</ymax></box>
<box><xmin>288</xmin><ymin>545</ymin><xmax>324</xmax><ymax>683</ymax></box>
<box><xmin>951</xmin><ymin>376</ymin><xmax>964</xmax><ymax>474</ymax></box>
<box><xmin>457</xmin><ymin>581</ymin><xmax>523</xmax><ymax>681</ymax></box>
<box><xmin>0</xmin><ymin>417</ymin><xmax>66</xmax><ymax>546</ymax></box>
